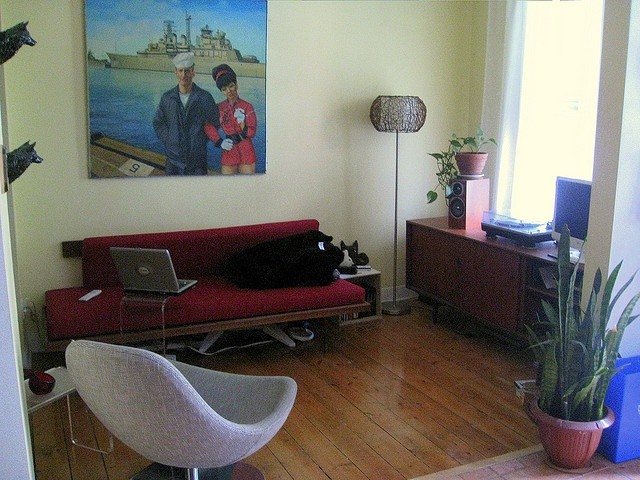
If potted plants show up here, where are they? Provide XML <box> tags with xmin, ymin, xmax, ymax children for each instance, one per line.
<box><xmin>425</xmin><ymin>126</ymin><xmax>500</xmax><ymax>209</ymax></box>
<box><xmin>516</xmin><ymin>223</ymin><xmax>640</xmax><ymax>469</ymax></box>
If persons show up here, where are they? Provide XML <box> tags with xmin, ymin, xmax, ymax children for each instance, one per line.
<box><xmin>203</xmin><ymin>64</ymin><xmax>258</xmax><ymax>174</ymax></box>
<box><xmin>153</xmin><ymin>53</ymin><xmax>220</xmax><ymax>175</ymax></box>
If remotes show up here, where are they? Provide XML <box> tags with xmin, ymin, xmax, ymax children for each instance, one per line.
<box><xmin>77</xmin><ymin>289</ymin><xmax>103</xmax><ymax>302</ymax></box>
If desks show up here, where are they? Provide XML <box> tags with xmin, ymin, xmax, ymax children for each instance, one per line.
<box><xmin>405</xmin><ymin>214</ymin><xmax>587</xmax><ymax>343</ymax></box>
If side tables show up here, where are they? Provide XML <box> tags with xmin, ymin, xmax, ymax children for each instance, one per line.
<box><xmin>23</xmin><ymin>367</ymin><xmax>115</xmax><ymax>456</ymax></box>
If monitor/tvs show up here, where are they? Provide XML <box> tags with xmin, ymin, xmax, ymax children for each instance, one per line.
<box><xmin>548</xmin><ymin>176</ymin><xmax>592</xmax><ymax>264</ymax></box>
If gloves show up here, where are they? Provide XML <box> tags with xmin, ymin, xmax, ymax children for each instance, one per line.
<box><xmin>221</xmin><ymin>139</ymin><xmax>234</xmax><ymax>151</ymax></box>
<box><xmin>233</xmin><ymin>108</ymin><xmax>246</xmax><ymax>123</ymax></box>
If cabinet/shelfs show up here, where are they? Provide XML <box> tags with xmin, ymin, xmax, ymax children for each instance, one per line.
<box><xmin>339</xmin><ymin>262</ymin><xmax>383</xmax><ymax>327</ymax></box>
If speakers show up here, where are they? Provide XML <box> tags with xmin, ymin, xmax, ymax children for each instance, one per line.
<box><xmin>449</xmin><ymin>177</ymin><xmax>491</xmax><ymax>231</ymax></box>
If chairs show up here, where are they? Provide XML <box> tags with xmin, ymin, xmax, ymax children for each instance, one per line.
<box><xmin>65</xmin><ymin>341</ymin><xmax>298</xmax><ymax>480</ymax></box>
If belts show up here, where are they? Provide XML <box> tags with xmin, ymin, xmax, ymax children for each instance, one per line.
<box><xmin>226</xmin><ymin>133</ymin><xmax>245</xmax><ymax>143</ymax></box>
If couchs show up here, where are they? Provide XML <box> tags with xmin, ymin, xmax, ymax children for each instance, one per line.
<box><xmin>44</xmin><ymin>217</ymin><xmax>371</xmax><ymax>354</ymax></box>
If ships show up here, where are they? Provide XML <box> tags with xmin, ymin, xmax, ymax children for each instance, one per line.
<box><xmin>105</xmin><ymin>10</ymin><xmax>267</xmax><ymax>79</ymax></box>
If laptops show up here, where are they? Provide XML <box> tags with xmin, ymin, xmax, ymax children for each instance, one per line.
<box><xmin>109</xmin><ymin>245</ymin><xmax>199</xmax><ymax>294</ymax></box>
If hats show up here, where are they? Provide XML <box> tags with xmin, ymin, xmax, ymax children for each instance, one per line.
<box><xmin>173</xmin><ymin>52</ymin><xmax>194</xmax><ymax>69</ymax></box>
<box><xmin>212</xmin><ymin>63</ymin><xmax>236</xmax><ymax>81</ymax></box>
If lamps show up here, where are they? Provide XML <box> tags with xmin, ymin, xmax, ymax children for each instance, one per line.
<box><xmin>369</xmin><ymin>95</ymin><xmax>427</xmax><ymax>317</ymax></box>
